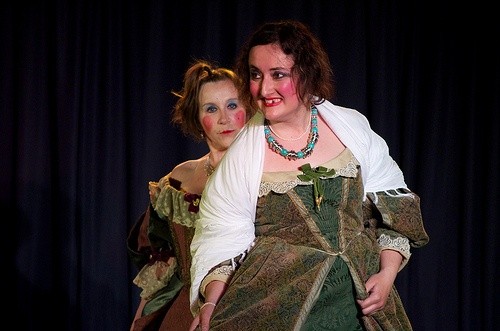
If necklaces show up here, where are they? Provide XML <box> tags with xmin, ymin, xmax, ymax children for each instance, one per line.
<box><xmin>267</xmin><ymin>106</ymin><xmax>312</xmax><ymax>141</ymax></box>
<box><xmin>264</xmin><ymin>106</ymin><xmax>318</xmax><ymax>160</ymax></box>
<box><xmin>204</xmin><ymin>155</ymin><xmax>217</xmax><ymax>176</ymax></box>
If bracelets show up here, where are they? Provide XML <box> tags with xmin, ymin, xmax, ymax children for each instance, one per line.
<box><xmin>200</xmin><ymin>303</ymin><xmax>217</xmax><ymax>309</ymax></box>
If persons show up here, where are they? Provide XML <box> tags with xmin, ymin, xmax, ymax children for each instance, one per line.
<box><xmin>125</xmin><ymin>63</ymin><xmax>255</xmax><ymax>331</ymax></box>
<box><xmin>189</xmin><ymin>18</ymin><xmax>428</xmax><ymax>331</ymax></box>
